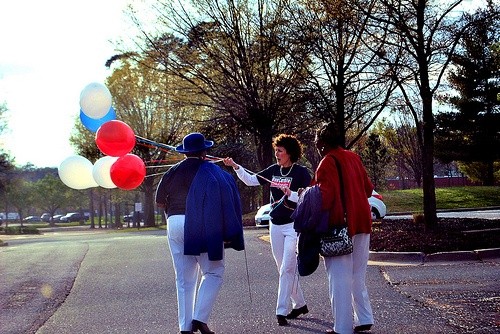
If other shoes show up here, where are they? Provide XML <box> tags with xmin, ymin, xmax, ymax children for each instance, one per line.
<box><xmin>325</xmin><ymin>329</ymin><xmax>340</xmax><ymax>334</ymax></box>
<box><xmin>277</xmin><ymin>315</ymin><xmax>287</xmax><ymax>326</ymax></box>
<box><xmin>287</xmin><ymin>305</ymin><xmax>309</xmax><ymax>319</ymax></box>
<box><xmin>354</xmin><ymin>324</ymin><xmax>375</xmax><ymax>334</ymax></box>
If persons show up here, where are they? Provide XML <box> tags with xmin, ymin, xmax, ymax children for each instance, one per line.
<box><xmin>156</xmin><ymin>132</ymin><xmax>246</xmax><ymax>334</ymax></box>
<box><xmin>293</xmin><ymin>122</ymin><xmax>373</xmax><ymax>334</ymax></box>
<box><xmin>222</xmin><ymin>134</ymin><xmax>313</xmax><ymax>326</ymax></box>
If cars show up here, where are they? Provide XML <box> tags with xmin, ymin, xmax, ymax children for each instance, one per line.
<box><xmin>254</xmin><ymin>186</ymin><xmax>387</xmax><ymax>231</ymax></box>
<box><xmin>49</xmin><ymin>214</ymin><xmax>63</xmax><ymax>223</ymax></box>
<box><xmin>59</xmin><ymin>212</ymin><xmax>90</xmax><ymax>222</ymax></box>
<box><xmin>122</xmin><ymin>210</ymin><xmax>144</xmax><ymax>222</ymax></box>
<box><xmin>23</xmin><ymin>215</ymin><xmax>40</xmax><ymax>223</ymax></box>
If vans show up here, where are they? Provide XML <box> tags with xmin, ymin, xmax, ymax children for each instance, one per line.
<box><xmin>0</xmin><ymin>213</ymin><xmax>20</xmax><ymax>223</ymax></box>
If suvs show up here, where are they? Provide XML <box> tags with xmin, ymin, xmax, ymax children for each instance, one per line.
<box><xmin>40</xmin><ymin>213</ymin><xmax>57</xmax><ymax>222</ymax></box>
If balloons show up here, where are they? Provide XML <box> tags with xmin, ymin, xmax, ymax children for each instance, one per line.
<box><xmin>95</xmin><ymin>120</ymin><xmax>135</xmax><ymax>157</ymax></box>
<box><xmin>92</xmin><ymin>155</ymin><xmax>119</xmax><ymax>188</ymax></box>
<box><xmin>58</xmin><ymin>155</ymin><xmax>99</xmax><ymax>190</ymax></box>
<box><xmin>79</xmin><ymin>107</ymin><xmax>116</xmax><ymax>135</ymax></box>
<box><xmin>110</xmin><ymin>154</ymin><xmax>146</xmax><ymax>190</ymax></box>
<box><xmin>80</xmin><ymin>82</ymin><xmax>113</xmax><ymax>120</ymax></box>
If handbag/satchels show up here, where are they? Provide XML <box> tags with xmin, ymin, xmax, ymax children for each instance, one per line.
<box><xmin>319</xmin><ymin>225</ymin><xmax>354</xmax><ymax>257</ymax></box>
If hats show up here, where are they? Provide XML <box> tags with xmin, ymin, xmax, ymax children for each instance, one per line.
<box><xmin>175</xmin><ymin>132</ymin><xmax>214</xmax><ymax>153</ymax></box>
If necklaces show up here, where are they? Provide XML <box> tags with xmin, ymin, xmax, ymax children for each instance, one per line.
<box><xmin>280</xmin><ymin>162</ymin><xmax>293</xmax><ymax>176</ymax></box>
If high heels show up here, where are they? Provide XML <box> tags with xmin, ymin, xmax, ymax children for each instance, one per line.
<box><xmin>192</xmin><ymin>320</ymin><xmax>214</xmax><ymax>334</ymax></box>
<box><xmin>180</xmin><ymin>331</ymin><xmax>191</xmax><ymax>334</ymax></box>
